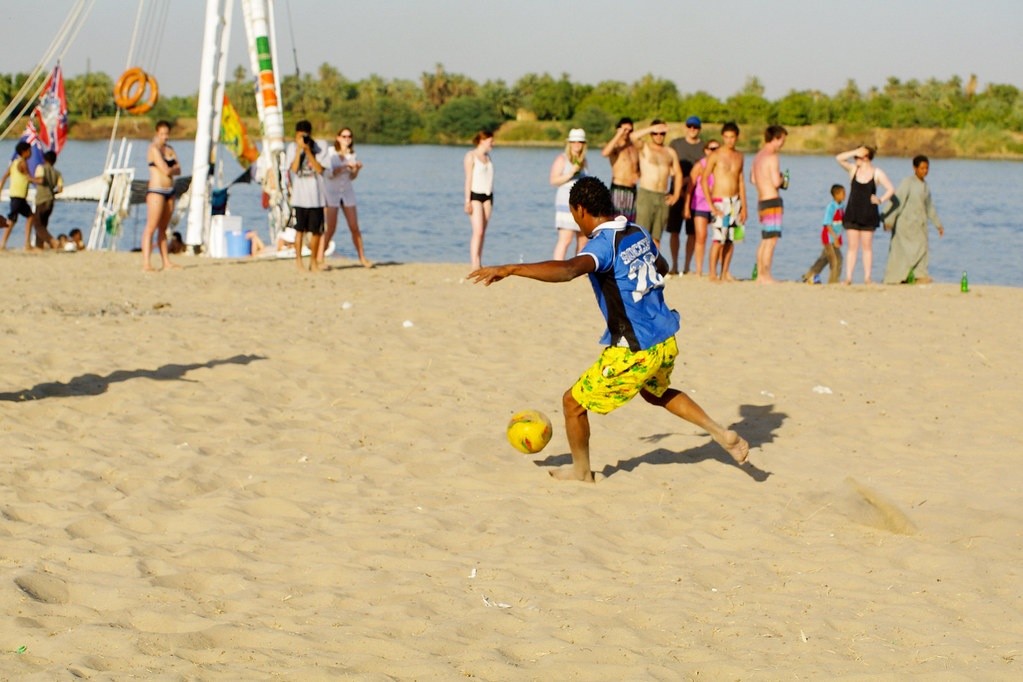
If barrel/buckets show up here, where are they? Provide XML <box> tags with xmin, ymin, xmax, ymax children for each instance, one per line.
<box><xmin>226</xmin><ymin>231</ymin><xmax>251</xmax><ymax>256</ymax></box>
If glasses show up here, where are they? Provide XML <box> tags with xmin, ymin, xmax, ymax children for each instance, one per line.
<box><xmin>686</xmin><ymin>123</ymin><xmax>702</xmax><ymax>129</ymax></box>
<box><xmin>653</xmin><ymin>132</ymin><xmax>665</xmax><ymax>136</ymax></box>
<box><xmin>706</xmin><ymin>148</ymin><xmax>717</xmax><ymax>150</ymax></box>
<box><xmin>340</xmin><ymin>135</ymin><xmax>353</xmax><ymax>139</ymax></box>
<box><xmin>570</xmin><ymin>141</ymin><xmax>585</xmax><ymax>144</ymax></box>
<box><xmin>854</xmin><ymin>156</ymin><xmax>861</xmax><ymax>158</ymax></box>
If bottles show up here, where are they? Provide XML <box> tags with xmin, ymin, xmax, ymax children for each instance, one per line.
<box><xmin>574</xmin><ymin>155</ymin><xmax>580</xmax><ymax>176</ymax></box>
<box><xmin>961</xmin><ymin>271</ymin><xmax>968</xmax><ymax>292</ymax></box>
<box><xmin>782</xmin><ymin>169</ymin><xmax>789</xmax><ymax>189</ymax></box>
<box><xmin>909</xmin><ymin>266</ymin><xmax>914</xmax><ymax>282</ymax></box>
<box><xmin>753</xmin><ymin>263</ymin><xmax>757</xmax><ymax>280</ymax></box>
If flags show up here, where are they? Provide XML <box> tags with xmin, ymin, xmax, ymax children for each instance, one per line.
<box><xmin>212</xmin><ymin>94</ymin><xmax>260</xmax><ymax>193</ymax></box>
<box><xmin>11</xmin><ymin>63</ymin><xmax>69</xmax><ymax>189</ymax></box>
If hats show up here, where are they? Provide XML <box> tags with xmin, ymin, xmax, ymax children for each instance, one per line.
<box><xmin>686</xmin><ymin>116</ymin><xmax>701</xmax><ymax>125</ymax></box>
<box><xmin>567</xmin><ymin>128</ymin><xmax>586</xmax><ymax>142</ymax></box>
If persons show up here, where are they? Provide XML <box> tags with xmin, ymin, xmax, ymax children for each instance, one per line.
<box><xmin>682</xmin><ymin>139</ymin><xmax>722</xmax><ymax>281</ymax></box>
<box><xmin>668</xmin><ymin>117</ymin><xmax>708</xmax><ymax>275</ymax></box>
<box><xmin>464</xmin><ymin>129</ymin><xmax>494</xmax><ymax>272</ymax></box>
<box><xmin>835</xmin><ymin>143</ymin><xmax>895</xmax><ymax>286</ymax></box>
<box><xmin>550</xmin><ymin>128</ymin><xmax>588</xmax><ymax>261</ymax></box>
<box><xmin>166</xmin><ymin>231</ymin><xmax>187</xmax><ymax>254</ymax></box>
<box><xmin>700</xmin><ymin>121</ymin><xmax>747</xmax><ymax>281</ymax></box>
<box><xmin>246</xmin><ymin>231</ymin><xmax>266</xmax><ymax>257</ymax></box>
<box><xmin>749</xmin><ymin>125</ymin><xmax>788</xmax><ymax>281</ymax></box>
<box><xmin>57</xmin><ymin>228</ymin><xmax>85</xmax><ymax>253</ymax></box>
<box><xmin>316</xmin><ymin>127</ymin><xmax>376</xmax><ymax>271</ymax></box>
<box><xmin>467</xmin><ymin>174</ymin><xmax>749</xmax><ymax>484</ymax></box>
<box><xmin>802</xmin><ymin>184</ymin><xmax>845</xmax><ymax>284</ymax></box>
<box><xmin>602</xmin><ymin>117</ymin><xmax>640</xmax><ymax>223</ymax></box>
<box><xmin>629</xmin><ymin>119</ymin><xmax>682</xmax><ymax>250</ymax></box>
<box><xmin>879</xmin><ymin>154</ymin><xmax>943</xmax><ymax>285</ymax></box>
<box><xmin>286</xmin><ymin>120</ymin><xmax>327</xmax><ymax>273</ymax></box>
<box><xmin>0</xmin><ymin>140</ymin><xmax>48</xmax><ymax>253</ymax></box>
<box><xmin>27</xmin><ymin>150</ymin><xmax>63</xmax><ymax>253</ymax></box>
<box><xmin>141</xmin><ymin>120</ymin><xmax>183</xmax><ymax>273</ymax></box>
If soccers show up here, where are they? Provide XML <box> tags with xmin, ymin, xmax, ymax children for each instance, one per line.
<box><xmin>506</xmin><ymin>410</ymin><xmax>553</xmax><ymax>455</ymax></box>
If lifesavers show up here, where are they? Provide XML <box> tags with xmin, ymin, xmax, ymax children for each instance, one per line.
<box><xmin>113</xmin><ymin>67</ymin><xmax>146</xmax><ymax>108</ymax></box>
<box><xmin>121</xmin><ymin>72</ymin><xmax>158</xmax><ymax>116</ymax></box>
